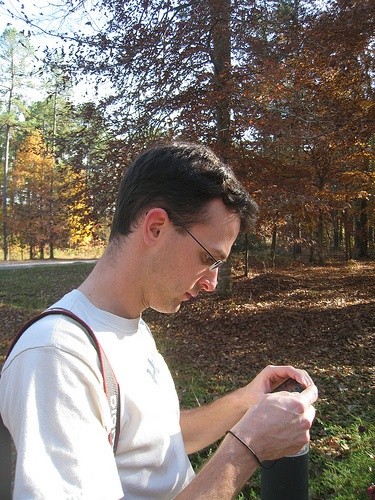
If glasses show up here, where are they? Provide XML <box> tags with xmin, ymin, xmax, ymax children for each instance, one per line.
<box><xmin>180</xmin><ymin>224</ymin><xmax>224</xmax><ymax>271</ymax></box>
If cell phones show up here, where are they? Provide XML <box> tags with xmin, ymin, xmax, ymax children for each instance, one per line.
<box><xmin>271</xmin><ymin>375</ymin><xmax>306</xmax><ymax>399</ymax></box>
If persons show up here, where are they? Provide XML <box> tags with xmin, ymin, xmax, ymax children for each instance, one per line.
<box><xmin>0</xmin><ymin>141</ymin><xmax>321</xmax><ymax>500</ymax></box>
